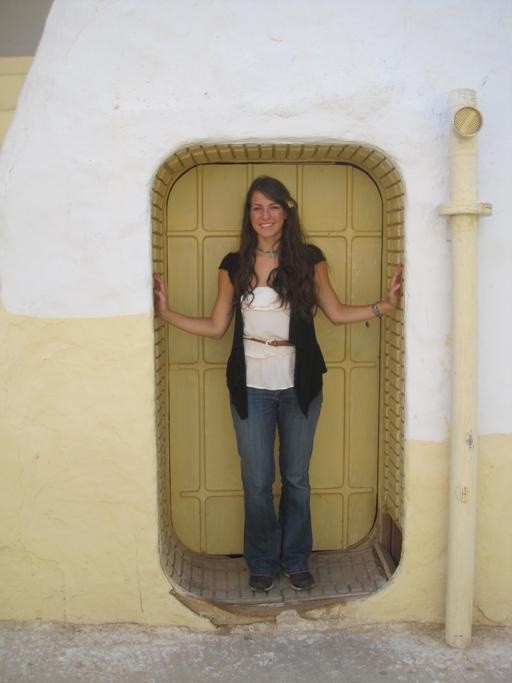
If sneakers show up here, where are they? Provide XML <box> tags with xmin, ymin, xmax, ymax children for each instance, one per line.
<box><xmin>281</xmin><ymin>566</ymin><xmax>316</xmax><ymax>591</ymax></box>
<box><xmin>248</xmin><ymin>570</ymin><xmax>279</xmax><ymax>591</ymax></box>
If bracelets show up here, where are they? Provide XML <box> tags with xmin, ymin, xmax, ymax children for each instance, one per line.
<box><xmin>373</xmin><ymin>303</ymin><xmax>382</xmax><ymax>319</ymax></box>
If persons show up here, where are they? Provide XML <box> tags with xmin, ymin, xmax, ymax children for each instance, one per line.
<box><xmin>154</xmin><ymin>173</ymin><xmax>404</xmax><ymax>592</ymax></box>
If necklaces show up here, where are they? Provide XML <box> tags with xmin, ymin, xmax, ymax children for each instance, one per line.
<box><xmin>255</xmin><ymin>244</ymin><xmax>281</xmax><ymax>256</ymax></box>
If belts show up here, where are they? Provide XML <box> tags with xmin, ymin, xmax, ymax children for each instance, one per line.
<box><xmin>246</xmin><ymin>337</ymin><xmax>294</xmax><ymax>347</ymax></box>
